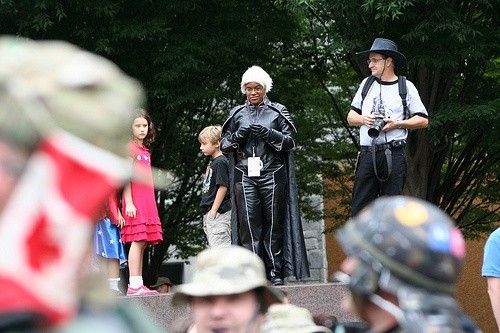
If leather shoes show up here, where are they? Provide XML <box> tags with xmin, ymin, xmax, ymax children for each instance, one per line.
<box><xmin>273</xmin><ymin>277</ymin><xmax>285</xmax><ymax>285</ymax></box>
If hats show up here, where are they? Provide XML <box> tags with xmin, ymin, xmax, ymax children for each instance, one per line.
<box><xmin>241</xmin><ymin>65</ymin><xmax>273</xmax><ymax>94</ymax></box>
<box><xmin>355</xmin><ymin>38</ymin><xmax>408</xmax><ymax>67</ymax></box>
<box><xmin>150</xmin><ymin>277</ymin><xmax>174</xmax><ymax>288</ymax></box>
<box><xmin>172</xmin><ymin>244</ymin><xmax>287</xmax><ymax>308</ymax></box>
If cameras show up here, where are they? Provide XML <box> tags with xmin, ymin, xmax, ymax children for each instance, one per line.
<box><xmin>368</xmin><ymin>114</ymin><xmax>390</xmax><ymax>138</ymax></box>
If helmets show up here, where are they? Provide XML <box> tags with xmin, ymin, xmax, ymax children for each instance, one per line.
<box><xmin>334</xmin><ymin>194</ymin><xmax>466</xmax><ymax>297</ymax></box>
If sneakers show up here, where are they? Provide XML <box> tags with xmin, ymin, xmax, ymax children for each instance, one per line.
<box><xmin>127</xmin><ymin>284</ymin><xmax>160</xmax><ymax>297</ymax></box>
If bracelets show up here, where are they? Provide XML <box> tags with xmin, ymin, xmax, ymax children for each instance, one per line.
<box><xmin>397</xmin><ymin>119</ymin><xmax>404</xmax><ymax>129</ymax></box>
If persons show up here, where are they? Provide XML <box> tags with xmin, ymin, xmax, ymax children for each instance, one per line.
<box><xmin>332</xmin><ymin>39</ymin><xmax>430</xmax><ymax>283</ymax></box>
<box><xmin>198</xmin><ymin>124</ymin><xmax>232</xmax><ymax>246</ymax></box>
<box><xmin>0</xmin><ymin>35</ymin><xmax>170</xmax><ymax>332</ymax></box>
<box><xmin>482</xmin><ymin>226</ymin><xmax>500</xmax><ymax>328</ymax></box>
<box><xmin>172</xmin><ymin>244</ymin><xmax>283</xmax><ymax>333</ymax></box>
<box><xmin>121</xmin><ymin>108</ymin><xmax>165</xmax><ymax>296</ymax></box>
<box><xmin>264</xmin><ymin>286</ymin><xmax>351</xmax><ymax>333</ymax></box>
<box><xmin>93</xmin><ymin>192</ymin><xmax>128</xmax><ymax>298</ymax></box>
<box><xmin>218</xmin><ymin>64</ymin><xmax>296</xmax><ymax>287</ymax></box>
<box><xmin>333</xmin><ymin>196</ymin><xmax>469</xmax><ymax>332</ymax></box>
<box><xmin>152</xmin><ymin>277</ymin><xmax>174</xmax><ymax>294</ymax></box>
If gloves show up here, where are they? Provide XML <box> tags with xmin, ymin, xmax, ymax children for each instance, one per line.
<box><xmin>248</xmin><ymin>124</ymin><xmax>271</xmax><ymax>139</ymax></box>
<box><xmin>235</xmin><ymin>126</ymin><xmax>250</xmax><ymax>141</ymax></box>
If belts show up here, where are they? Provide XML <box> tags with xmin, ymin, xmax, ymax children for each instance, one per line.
<box><xmin>360</xmin><ymin>140</ymin><xmax>405</xmax><ymax>152</ymax></box>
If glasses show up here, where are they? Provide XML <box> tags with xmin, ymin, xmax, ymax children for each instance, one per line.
<box><xmin>245</xmin><ymin>87</ymin><xmax>263</xmax><ymax>92</ymax></box>
<box><xmin>367</xmin><ymin>58</ymin><xmax>387</xmax><ymax>64</ymax></box>
